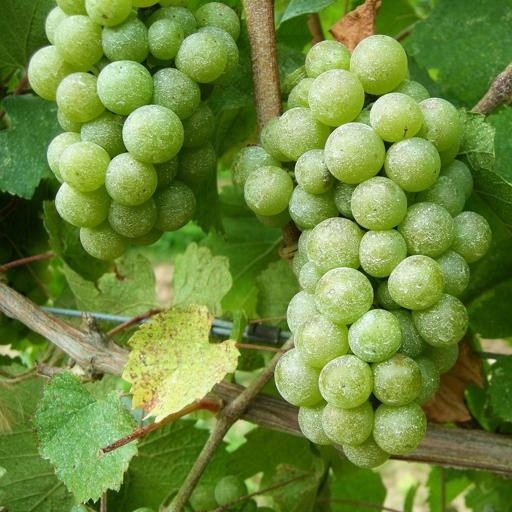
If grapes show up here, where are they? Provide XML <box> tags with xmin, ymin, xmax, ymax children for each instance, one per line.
<box><xmin>26</xmin><ymin>0</ymin><xmax>242</xmax><ymax>261</ymax></box>
<box><xmin>132</xmin><ymin>476</ymin><xmax>277</xmax><ymax>512</ymax></box>
<box><xmin>231</xmin><ymin>35</ymin><xmax>492</xmax><ymax>468</ymax></box>
